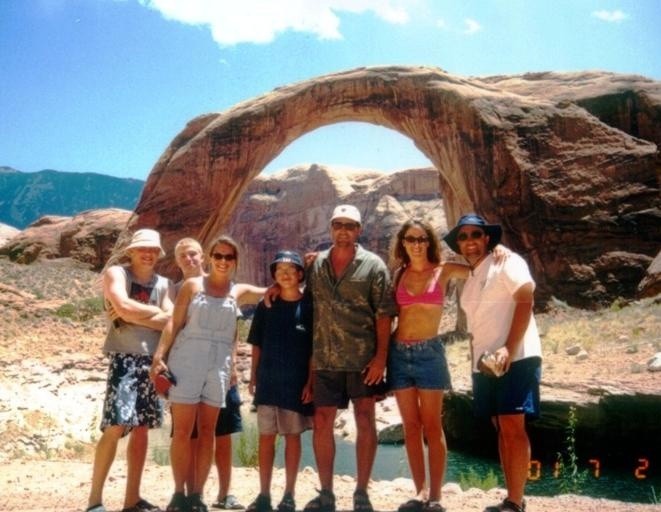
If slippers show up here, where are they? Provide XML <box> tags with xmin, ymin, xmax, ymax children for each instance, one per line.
<box><xmin>399</xmin><ymin>498</ymin><xmax>444</xmax><ymax>511</ymax></box>
<box><xmin>121</xmin><ymin>492</ymin><xmax>244</xmax><ymax>511</ymax></box>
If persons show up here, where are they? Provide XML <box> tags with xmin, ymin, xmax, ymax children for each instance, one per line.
<box><xmin>170</xmin><ymin>237</ymin><xmax>245</xmax><ymax>510</ymax></box>
<box><xmin>247</xmin><ymin>250</ymin><xmax>315</xmax><ymax>512</ymax></box>
<box><xmin>387</xmin><ymin>218</ymin><xmax>511</xmax><ymax>512</ymax></box>
<box><xmin>264</xmin><ymin>205</ymin><xmax>396</xmax><ymax>512</ymax></box>
<box><xmin>149</xmin><ymin>237</ymin><xmax>318</xmax><ymax>512</ymax></box>
<box><xmin>443</xmin><ymin>215</ymin><xmax>542</xmax><ymax>512</ymax></box>
<box><xmin>85</xmin><ymin>229</ymin><xmax>176</xmax><ymax>512</ymax></box>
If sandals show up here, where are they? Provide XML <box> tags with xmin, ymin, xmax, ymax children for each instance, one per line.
<box><xmin>248</xmin><ymin>491</ymin><xmax>376</xmax><ymax>512</ymax></box>
<box><xmin>485</xmin><ymin>496</ymin><xmax>528</xmax><ymax>512</ymax></box>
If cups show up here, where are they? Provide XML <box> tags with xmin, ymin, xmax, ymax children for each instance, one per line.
<box><xmin>480</xmin><ymin>349</ymin><xmax>503</xmax><ymax>378</ymax></box>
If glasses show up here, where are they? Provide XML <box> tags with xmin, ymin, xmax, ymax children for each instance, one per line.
<box><xmin>332</xmin><ymin>223</ymin><xmax>357</xmax><ymax>231</ymax></box>
<box><xmin>404</xmin><ymin>234</ymin><xmax>428</xmax><ymax>243</ymax></box>
<box><xmin>211</xmin><ymin>251</ymin><xmax>235</xmax><ymax>260</ymax></box>
<box><xmin>457</xmin><ymin>231</ymin><xmax>482</xmax><ymax>241</ymax></box>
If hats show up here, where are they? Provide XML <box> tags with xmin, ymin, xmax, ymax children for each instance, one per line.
<box><xmin>125</xmin><ymin>229</ymin><xmax>165</xmax><ymax>259</ymax></box>
<box><xmin>330</xmin><ymin>205</ymin><xmax>361</xmax><ymax>230</ymax></box>
<box><xmin>443</xmin><ymin>215</ymin><xmax>502</xmax><ymax>255</ymax></box>
<box><xmin>270</xmin><ymin>251</ymin><xmax>305</xmax><ymax>283</ymax></box>
